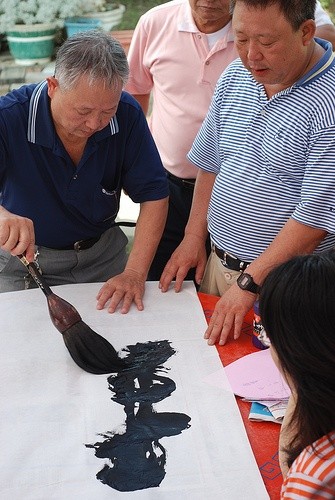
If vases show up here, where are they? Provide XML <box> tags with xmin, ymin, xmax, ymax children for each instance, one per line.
<box><xmin>63</xmin><ymin>3</ymin><xmax>126</xmax><ymax>42</ymax></box>
<box><xmin>5</xmin><ymin>18</ymin><xmax>60</xmax><ymax>66</ymax></box>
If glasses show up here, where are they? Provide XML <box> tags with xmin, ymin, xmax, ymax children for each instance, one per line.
<box><xmin>259</xmin><ymin>328</ymin><xmax>273</xmax><ymax>347</ymax></box>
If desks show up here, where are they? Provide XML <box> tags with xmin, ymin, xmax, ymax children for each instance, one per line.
<box><xmin>0</xmin><ymin>278</ymin><xmax>299</xmax><ymax>500</ymax></box>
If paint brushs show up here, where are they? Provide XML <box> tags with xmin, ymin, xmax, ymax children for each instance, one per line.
<box><xmin>11</xmin><ymin>242</ymin><xmax>133</xmax><ymax>374</ymax></box>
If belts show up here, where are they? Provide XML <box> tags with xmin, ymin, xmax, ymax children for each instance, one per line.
<box><xmin>34</xmin><ymin>218</ymin><xmax>114</xmax><ymax>251</ymax></box>
<box><xmin>210</xmin><ymin>238</ymin><xmax>251</xmax><ymax>272</ymax></box>
<box><xmin>164</xmin><ymin>168</ymin><xmax>195</xmax><ymax>190</ymax></box>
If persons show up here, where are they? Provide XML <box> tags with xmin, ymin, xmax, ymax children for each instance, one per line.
<box><xmin>314</xmin><ymin>0</ymin><xmax>335</xmax><ymax>52</ymax></box>
<box><xmin>0</xmin><ymin>30</ymin><xmax>169</xmax><ymax>314</ymax></box>
<box><xmin>158</xmin><ymin>0</ymin><xmax>335</xmax><ymax>346</ymax></box>
<box><xmin>259</xmin><ymin>255</ymin><xmax>335</xmax><ymax>500</ymax></box>
<box><xmin>123</xmin><ymin>0</ymin><xmax>240</xmax><ymax>292</ymax></box>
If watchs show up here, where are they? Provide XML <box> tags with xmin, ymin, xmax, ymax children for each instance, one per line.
<box><xmin>237</xmin><ymin>273</ymin><xmax>261</xmax><ymax>295</ymax></box>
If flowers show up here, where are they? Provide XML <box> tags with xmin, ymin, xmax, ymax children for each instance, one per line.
<box><xmin>0</xmin><ymin>0</ymin><xmax>107</xmax><ymax>35</ymax></box>
